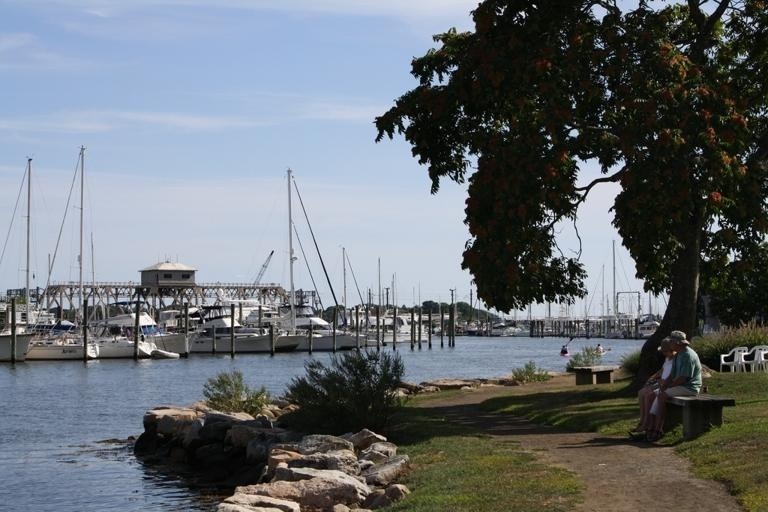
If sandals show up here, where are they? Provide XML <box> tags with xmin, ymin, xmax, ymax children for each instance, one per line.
<box><xmin>628</xmin><ymin>422</ymin><xmax>665</xmax><ymax>443</ymax></box>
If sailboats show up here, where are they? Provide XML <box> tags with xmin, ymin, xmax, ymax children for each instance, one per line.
<box><xmin>431</xmin><ymin>240</ymin><xmax>669</xmax><ymax>338</ymax></box>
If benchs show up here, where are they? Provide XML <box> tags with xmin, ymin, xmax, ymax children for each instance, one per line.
<box><xmin>666</xmin><ymin>394</ymin><xmax>735</xmax><ymax>441</ymax></box>
<box><xmin>572</xmin><ymin>365</ymin><xmax>614</xmax><ymax>386</ymax></box>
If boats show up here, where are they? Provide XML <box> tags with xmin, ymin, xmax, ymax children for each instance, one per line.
<box><xmin>592</xmin><ymin>349</ymin><xmax>608</xmax><ymax>356</ymax></box>
<box><xmin>560</xmin><ymin>345</ymin><xmax>572</xmax><ymax>360</ymax></box>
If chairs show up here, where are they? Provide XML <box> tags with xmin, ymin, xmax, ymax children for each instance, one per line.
<box><xmin>720</xmin><ymin>345</ymin><xmax>768</xmax><ymax>374</ymax></box>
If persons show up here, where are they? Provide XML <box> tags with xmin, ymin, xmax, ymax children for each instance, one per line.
<box><xmin>561</xmin><ymin>346</ymin><xmax>568</xmax><ymax>354</ymax></box>
<box><xmin>631</xmin><ymin>331</ymin><xmax>702</xmax><ymax>432</ymax></box>
<box><xmin>596</xmin><ymin>344</ymin><xmax>603</xmax><ymax>353</ymax></box>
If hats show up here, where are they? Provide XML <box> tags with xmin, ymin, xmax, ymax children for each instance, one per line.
<box><xmin>657</xmin><ymin>330</ymin><xmax>690</xmax><ymax>352</ymax></box>
